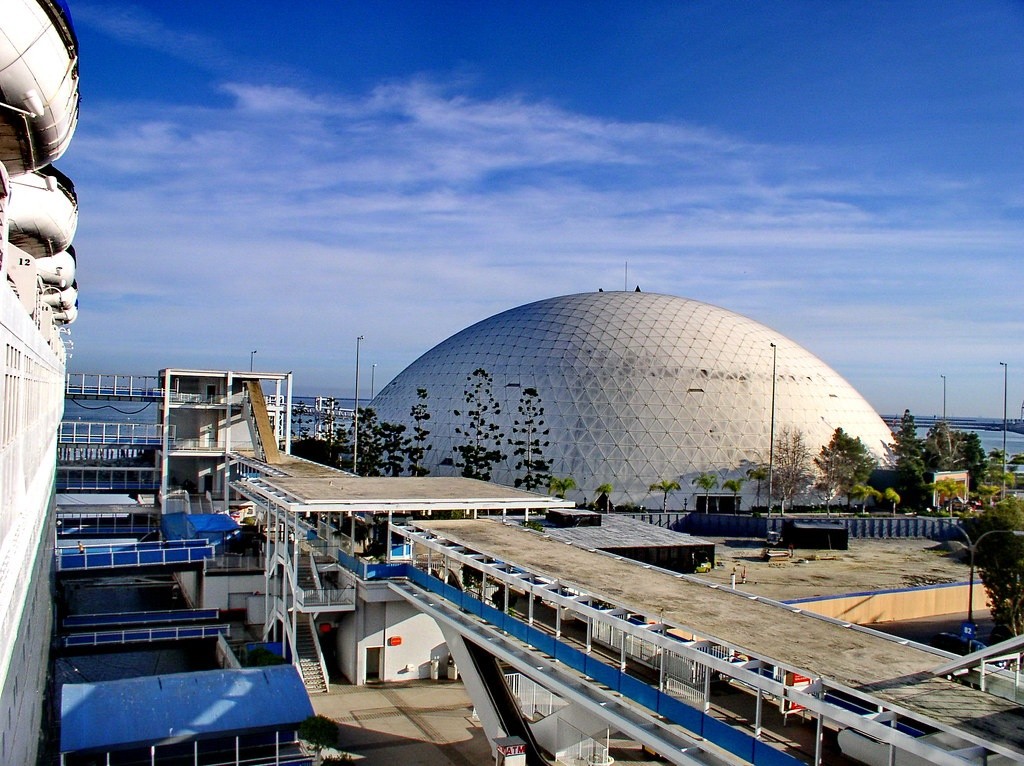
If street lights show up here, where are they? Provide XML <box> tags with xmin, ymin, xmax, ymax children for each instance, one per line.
<box><xmin>926</xmin><ymin>517</ymin><xmax>1024</xmax><ymax>654</ymax></box>
<box><xmin>371</xmin><ymin>363</ymin><xmax>377</xmax><ymax>400</ymax></box>
<box><xmin>999</xmin><ymin>362</ymin><xmax>1007</xmax><ymax>497</ymax></box>
<box><xmin>252</xmin><ymin>350</ymin><xmax>256</xmax><ymax>372</ymax></box>
<box><xmin>769</xmin><ymin>342</ymin><xmax>776</xmax><ymax>517</ymax></box>
<box><xmin>941</xmin><ymin>374</ymin><xmax>946</xmax><ymax>424</ymax></box>
<box><xmin>354</xmin><ymin>335</ymin><xmax>364</xmax><ymax>473</ymax></box>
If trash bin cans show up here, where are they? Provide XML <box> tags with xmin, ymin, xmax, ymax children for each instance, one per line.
<box><xmin>430</xmin><ymin>659</ymin><xmax>439</xmax><ymax>680</ymax></box>
